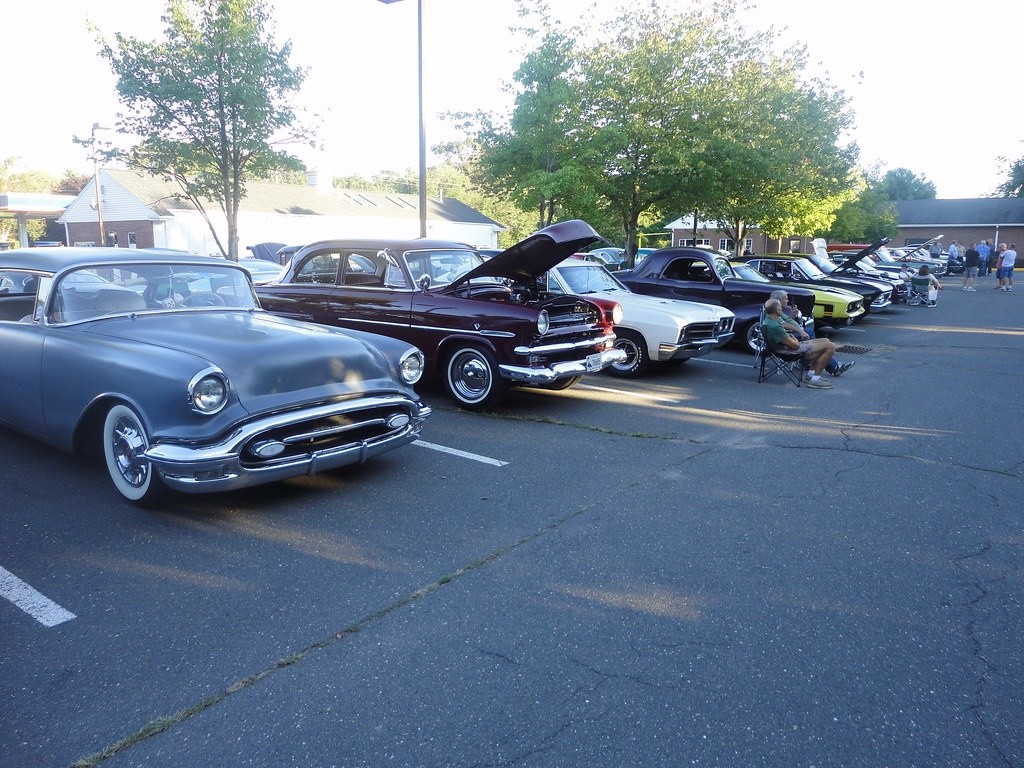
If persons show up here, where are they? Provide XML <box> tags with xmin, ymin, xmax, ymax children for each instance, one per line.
<box><xmin>743</xmin><ymin>246</ymin><xmax>751</xmax><ymax>255</ymax></box>
<box><xmin>900</xmin><ymin>264</ymin><xmax>913</xmax><ymax>296</ymax></box>
<box><xmin>975</xmin><ymin>239</ymin><xmax>995</xmax><ymax>277</ymax></box>
<box><xmin>761</xmin><ymin>299</ymin><xmax>836</xmax><ymax>388</ymax></box>
<box><xmin>962</xmin><ymin>242</ymin><xmax>981</xmax><ymax>291</ymax></box>
<box><xmin>770</xmin><ymin>290</ymin><xmax>855</xmax><ymax>376</ymax></box>
<box><xmin>929</xmin><ymin>240</ymin><xmax>943</xmax><ymax>258</ymax></box>
<box><xmin>949</xmin><ymin>239</ymin><xmax>966</xmax><ymax>261</ymax></box>
<box><xmin>913</xmin><ymin>265</ymin><xmax>942</xmax><ymax>305</ymax></box>
<box><xmin>994</xmin><ymin>242</ymin><xmax>1017</xmax><ymax>292</ymax></box>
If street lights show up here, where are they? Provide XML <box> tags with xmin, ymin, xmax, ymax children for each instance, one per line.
<box><xmin>378</xmin><ymin>0</ymin><xmax>429</xmax><ymax>275</ymax></box>
<box><xmin>90</xmin><ymin>122</ymin><xmax>113</xmax><ymax>246</ymax></box>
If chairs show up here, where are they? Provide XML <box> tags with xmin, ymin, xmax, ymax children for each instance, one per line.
<box><xmin>96</xmin><ymin>289</ymin><xmax>147</xmax><ymax>313</ymax></box>
<box><xmin>909</xmin><ymin>276</ymin><xmax>939</xmax><ymax>308</ymax></box>
<box><xmin>748</xmin><ymin>304</ymin><xmax>802</xmax><ymax>388</ymax></box>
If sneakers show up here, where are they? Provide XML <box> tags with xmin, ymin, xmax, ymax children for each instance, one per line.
<box><xmin>833</xmin><ymin>360</ymin><xmax>855</xmax><ymax>376</ymax></box>
<box><xmin>803</xmin><ymin>374</ymin><xmax>830</xmax><ymax>383</ymax></box>
<box><xmin>807</xmin><ymin>377</ymin><xmax>834</xmax><ymax>388</ymax></box>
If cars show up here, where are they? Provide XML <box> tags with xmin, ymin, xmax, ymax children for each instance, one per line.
<box><xmin>64</xmin><ymin>237</ymin><xmax>658</xmax><ymax>296</ymax></box>
<box><xmin>433</xmin><ymin>254</ymin><xmax>737</xmax><ymax>380</ymax></box>
<box><xmin>583</xmin><ymin>246</ymin><xmax>817</xmax><ymax>356</ymax></box>
<box><xmin>0</xmin><ymin>244</ymin><xmax>434</xmax><ymax>507</ymax></box>
<box><xmin>689</xmin><ymin>261</ymin><xmax>866</xmax><ymax>333</ymax></box>
<box><xmin>727</xmin><ymin>234</ymin><xmax>966</xmax><ymax>321</ymax></box>
<box><xmin>216</xmin><ymin>218</ymin><xmax>627</xmax><ymax>413</ymax></box>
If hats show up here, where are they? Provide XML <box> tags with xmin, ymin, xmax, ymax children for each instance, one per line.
<box><xmin>901</xmin><ymin>264</ymin><xmax>910</xmax><ymax>268</ymax></box>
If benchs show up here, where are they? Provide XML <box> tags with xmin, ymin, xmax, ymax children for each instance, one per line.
<box><xmin>302</xmin><ymin>271</ymin><xmax>381</xmax><ymax>285</ymax></box>
<box><xmin>0</xmin><ymin>291</ymin><xmax>113</xmax><ymax>319</ymax></box>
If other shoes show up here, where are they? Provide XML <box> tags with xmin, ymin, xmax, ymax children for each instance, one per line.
<box><xmin>967</xmin><ymin>287</ymin><xmax>976</xmax><ymax>291</ymax></box>
<box><xmin>999</xmin><ymin>288</ymin><xmax>1006</xmax><ymax>291</ymax></box>
<box><xmin>1006</xmin><ymin>288</ymin><xmax>1011</xmax><ymax>291</ymax></box>
<box><xmin>962</xmin><ymin>287</ymin><xmax>967</xmax><ymax>291</ymax></box>
